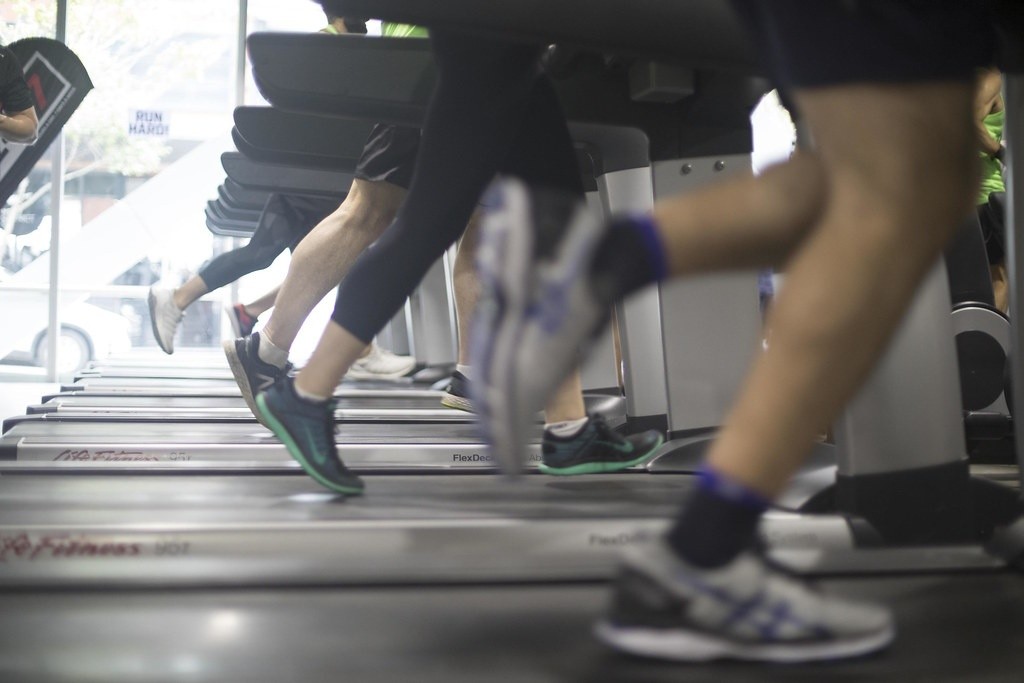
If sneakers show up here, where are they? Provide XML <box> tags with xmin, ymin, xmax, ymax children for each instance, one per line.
<box><xmin>222</xmin><ymin>331</ymin><xmax>294</xmax><ymax>433</ymax></box>
<box><xmin>350</xmin><ymin>343</ymin><xmax>418</xmax><ymax>378</ymax></box>
<box><xmin>224</xmin><ymin>304</ymin><xmax>259</xmax><ymax>339</ymax></box>
<box><xmin>467</xmin><ymin>174</ymin><xmax>612</xmax><ymax>480</ymax></box>
<box><xmin>252</xmin><ymin>375</ymin><xmax>366</xmax><ymax>496</ymax></box>
<box><xmin>147</xmin><ymin>285</ymin><xmax>186</xmax><ymax>355</ymax></box>
<box><xmin>440</xmin><ymin>369</ymin><xmax>473</xmax><ymax>417</ymax></box>
<box><xmin>592</xmin><ymin>548</ymin><xmax>898</xmax><ymax>665</ymax></box>
<box><xmin>539</xmin><ymin>413</ymin><xmax>665</xmax><ymax>475</ymax></box>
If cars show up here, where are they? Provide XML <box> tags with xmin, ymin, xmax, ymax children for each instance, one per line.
<box><xmin>1</xmin><ymin>264</ymin><xmax>134</xmax><ymax>382</ymax></box>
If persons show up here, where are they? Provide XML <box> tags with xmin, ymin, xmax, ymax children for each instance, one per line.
<box><xmin>146</xmin><ymin>0</ymin><xmax>1024</xmax><ymax>663</ymax></box>
<box><xmin>0</xmin><ymin>42</ymin><xmax>40</xmax><ymax>148</ymax></box>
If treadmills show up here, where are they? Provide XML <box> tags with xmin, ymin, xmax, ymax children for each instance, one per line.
<box><xmin>0</xmin><ymin>0</ymin><xmax>1024</xmax><ymax>683</ymax></box>
